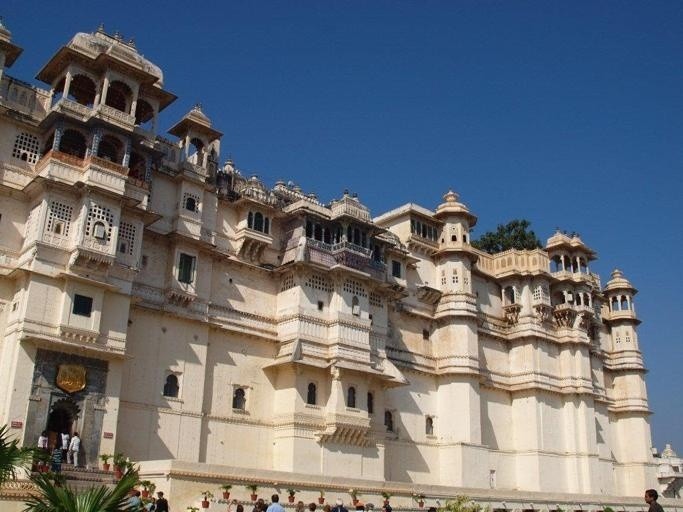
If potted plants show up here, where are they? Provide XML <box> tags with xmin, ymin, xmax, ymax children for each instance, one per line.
<box><xmin>99</xmin><ymin>451</ymin><xmax>151</xmax><ymax>498</ymax></box>
<box><xmin>201</xmin><ymin>483</ymin><xmax>426</xmax><ymax>508</ymax></box>
<box><xmin>32</xmin><ymin>448</ymin><xmax>52</xmax><ymax>473</ymax></box>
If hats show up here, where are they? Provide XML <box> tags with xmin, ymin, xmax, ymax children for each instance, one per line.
<box><xmin>156</xmin><ymin>491</ymin><xmax>165</xmax><ymax>495</ymax></box>
<box><xmin>336</xmin><ymin>496</ymin><xmax>343</xmax><ymax>506</ymax></box>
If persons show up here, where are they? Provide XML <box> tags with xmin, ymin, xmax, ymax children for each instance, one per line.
<box><xmin>37</xmin><ymin>430</ymin><xmax>48</xmax><ymax>466</ymax></box>
<box><xmin>50</xmin><ymin>443</ymin><xmax>61</xmax><ymax>480</ymax></box>
<box><xmin>47</xmin><ymin>425</ymin><xmax>58</xmax><ymax>455</ymax></box>
<box><xmin>59</xmin><ymin>428</ymin><xmax>69</xmax><ymax>462</ymax></box>
<box><xmin>142</xmin><ymin>497</ymin><xmax>157</xmax><ymax>512</ymax></box>
<box><xmin>236</xmin><ymin>494</ymin><xmax>392</xmax><ymax>512</ymax></box>
<box><xmin>154</xmin><ymin>491</ymin><xmax>168</xmax><ymax>512</ymax></box>
<box><xmin>127</xmin><ymin>490</ymin><xmax>143</xmax><ymax>512</ymax></box>
<box><xmin>66</xmin><ymin>431</ymin><xmax>80</xmax><ymax>467</ymax></box>
<box><xmin>644</xmin><ymin>488</ymin><xmax>664</xmax><ymax>511</ymax></box>
